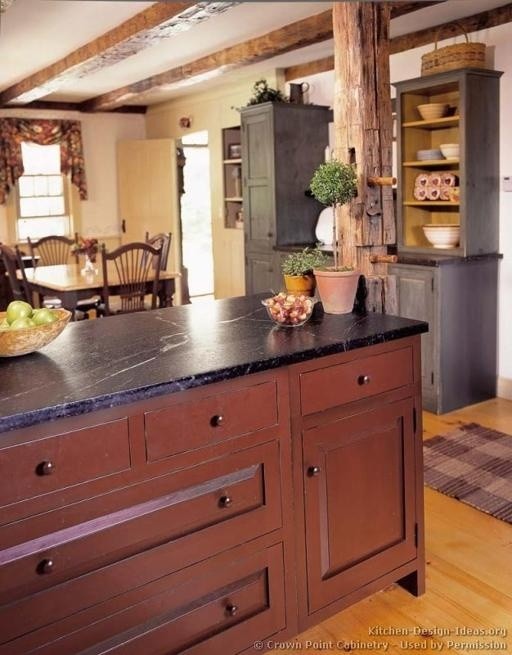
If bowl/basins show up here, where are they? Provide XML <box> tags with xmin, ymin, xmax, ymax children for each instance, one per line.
<box><xmin>262</xmin><ymin>297</ymin><xmax>320</xmax><ymax>326</ymax></box>
<box><xmin>0</xmin><ymin>308</ymin><xmax>74</xmax><ymax>356</ymax></box>
<box><xmin>439</xmin><ymin>143</ymin><xmax>459</xmax><ymax>159</ymax></box>
<box><xmin>417</xmin><ymin>104</ymin><xmax>448</xmax><ymax>119</ymax></box>
<box><xmin>422</xmin><ymin>224</ymin><xmax>460</xmax><ymax>249</ymax></box>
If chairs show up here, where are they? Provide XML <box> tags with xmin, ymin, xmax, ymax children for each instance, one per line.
<box><xmin>26</xmin><ymin>232</ymin><xmax>79</xmax><ymax>266</ymax></box>
<box><xmin>0</xmin><ymin>241</ymin><xmax>34</xmax><ymax>309</ymax></box>
<box><xmin>100</xmin><ymin>238</ymin><xmax>165</xmax><ymax>315</ymax></box>
<box><xmin>141</xmin><ymin>231</ymin><xmax>172</xmax><ymax>271</ymax></box>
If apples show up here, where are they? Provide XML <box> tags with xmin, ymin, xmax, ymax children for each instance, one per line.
<box><xmin>31</xmin><ymin>308</ymin><xmax>58</xmax><ymax>326</ymax></box>
<box><xmin>6</xmin><ymin>300</ymin><xmax>32</xmax><ymax>324</ymax></box>
<box><xmin>10</xmin><ymin>316</ymin><xmax>35</xmax><ymax>328</ymax></box>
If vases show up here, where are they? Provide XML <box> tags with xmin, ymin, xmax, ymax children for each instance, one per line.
<box><xmin>80</xmin><ymin>255</ymin><xmax>98</xmax><ymax>272</ymax></box>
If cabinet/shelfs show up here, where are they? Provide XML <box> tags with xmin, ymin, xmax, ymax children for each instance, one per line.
<box><xmin>387</xmin><ymin>262</ymin><xmax>499</xmax><ymax>415</ymax></box>
<box><xmin>239</xmin><ymin>101</ymin><xmax>331</xmax><ymax>248</ymax></box>
<box><xmin>219</xmin><ymin>124</ymin><xmax>245</xmax><ymax>232</ymax></box>
<box><xmin>0</xmin><ymin>369</ymin><xmax>297</xmax><ymax>654</ymax></box>
<box><xmin>242</xmin><ymin>248</ymin><xmax>277</xmax><ymax>295</ymax></box>
<box><xmin>289</xmin><ymin>334</ymin><xmax>427</xmax><ymax>634</ymax></box>
<box><xmin>389</xmin><ymin>66</ymin><xmax>505</xmax><ymax>259</ymax></box>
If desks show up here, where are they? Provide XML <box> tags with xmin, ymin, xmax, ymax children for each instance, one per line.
<box><xmin>5</xmin><ymin>262</ymin><xmax>183</xmax><ymax>321</ymax></box>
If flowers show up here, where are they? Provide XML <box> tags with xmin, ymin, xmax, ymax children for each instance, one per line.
<box><xmin>70</xmin><ymin>237</ymin><xmax>99</xmax><ymax>255</ymax></box>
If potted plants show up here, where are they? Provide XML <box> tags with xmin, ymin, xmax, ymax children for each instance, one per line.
<box><xmin>281</xmin><ymin>244</ymin><xmax>330</xmax><ymax>297</ymax></box>
<box><xmin>310</xmin><ymin>159</ymin><xmax>360</xmax><ymax>315</ymax></box>
